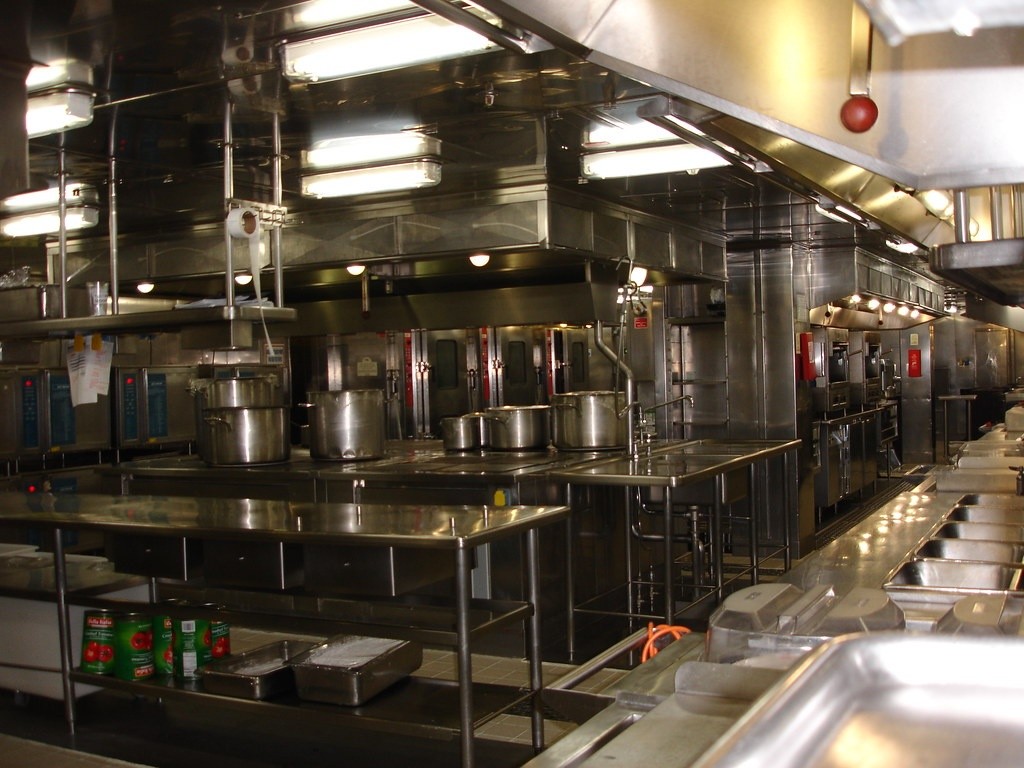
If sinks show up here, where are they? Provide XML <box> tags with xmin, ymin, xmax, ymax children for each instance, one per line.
<box><xmin>660</xmin><ymin>440</ymin><xmax>802</xmax><ymax>459</ymax></box>
<box><xmin>582</xmin><ymin>454</ymin><xmax>749</xmax><ymax>486</ymax></box>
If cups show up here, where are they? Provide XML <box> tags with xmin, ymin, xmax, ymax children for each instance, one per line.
<box><xmin>85</xmin><ymin>281</ymin><xmax>109</xmax><ymax>317</ymax></box>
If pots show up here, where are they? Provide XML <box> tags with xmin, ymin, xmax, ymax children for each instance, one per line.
<box><xmin>298</xmin><ymin>389</ymin><xmax>387</xmax><ymax>464</ymax></box>
<box><xmin>479</xmin><ymin>405</ymin><xmax>551</xmax><ymax>451</ymax></box>
<box><xmin>194</xmin><ymin>374</ymin><xmax>276</xmax><ymax>457</ymax></box>
<box><xmin>201</xmin><ymin>404</ymin><xmax>291</xmax><ymax>468</ymax></box>
<box><xmin>551</xmin><ymin>392</ymin><xmax>629</xmax><ymax>451</ymax></box>
<box><xmin>439</xmin><ymin>417</ymin><xmax>480</xmax><ymax>453</ymax></box>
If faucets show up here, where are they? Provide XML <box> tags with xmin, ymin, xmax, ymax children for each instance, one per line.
<box><xmin>645</xmin><ymin>395</ymin><xmax>695</xmax><ymax>413</ymax></box>
<box><xmin>618</xmin><ymin>402</ymin><xmax>645</xmax><ymax>447</ymax></box>
